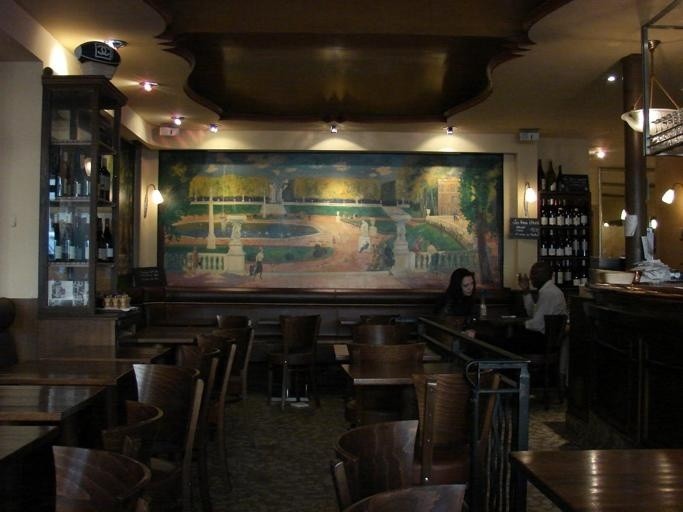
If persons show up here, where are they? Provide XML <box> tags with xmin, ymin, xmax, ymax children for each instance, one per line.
<box><xmin>251</xmin><ymin>245</ymin><xmax>266</xmax><ymax>283</ymax></box>
<box><xmin>491</xmin><ymin>260</ymin><xmax>568</xmax><ymax>377</ymax></box>
<box><xmin>433</xmin><ymin>265</ymin><xmax>484</xmax><ymax>363</ymax></box>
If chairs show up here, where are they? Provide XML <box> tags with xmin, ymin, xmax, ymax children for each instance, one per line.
<box><xmin>328</xmin><ymin>315</ymin><xmax>507</xmax><ymax>512</ymax></box>
<box><xmin>517</xmin><ymin>315</ymin><xmax>568</xmax><ymax>410</ymax></box>
<box><xmin>264</xmin><ymin>314</ymin><xmax>325</xmax><ymax>411</ymax></box>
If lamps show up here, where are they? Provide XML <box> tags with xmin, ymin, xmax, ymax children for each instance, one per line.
<box><xmin>441</xmin><ymin>126</ymin><xmax>457</xmax><ymax>135</ymax></box>
<box><xmin>105</xmin><ymin>39</ymin><xmax>220</xmax><ymax>134</ymax></box>
<box><xmin>621</xmin><ymin>40</ymin><xmax>683</xmax><ymax>134</ymax></box>
<box><xmin>331</xmin><ymin>124</ymin><xmax>340</xmax><ymax>133</ymax></box>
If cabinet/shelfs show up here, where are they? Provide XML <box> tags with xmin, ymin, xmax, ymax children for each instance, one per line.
<box><xmin>40</xmin><ymin>67</ymin><xmax>129</xmax><ymax>309</ymax></box>
<box><xmin>538</xmin><ymin>189</ymin><xmax>591</xmax><ymax>288</ymax></box>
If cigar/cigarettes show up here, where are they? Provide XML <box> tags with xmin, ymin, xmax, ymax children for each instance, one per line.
<box><xmin>515</xmin><ymin>273</ymin><xmax>520</xmax><ymax>278</ymax></box>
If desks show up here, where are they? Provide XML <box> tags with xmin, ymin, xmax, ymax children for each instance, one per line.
<box><xmin>590</xmin><ymin>282</ymin><xmax>683</xmax><ymax>449</ymax></box>
<box><xmin>1</xmin><ymin>320</ymin><xmax>196</xmax><ymax>511</ymax></box>
<box><xmin>508</xmin><ymin>450</ymin><xmax>681</xmax><ymax>510</ymax></box>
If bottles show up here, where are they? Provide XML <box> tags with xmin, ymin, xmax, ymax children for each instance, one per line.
<box><xmin>536</xmin><ymin>158</ymin><xmax>589</xmax><ymax>290</ymax></box>
<box><xmin>49</xmin><ymin>155</ymin><xmax>115</xmax><ymax>266</ymax></box>
